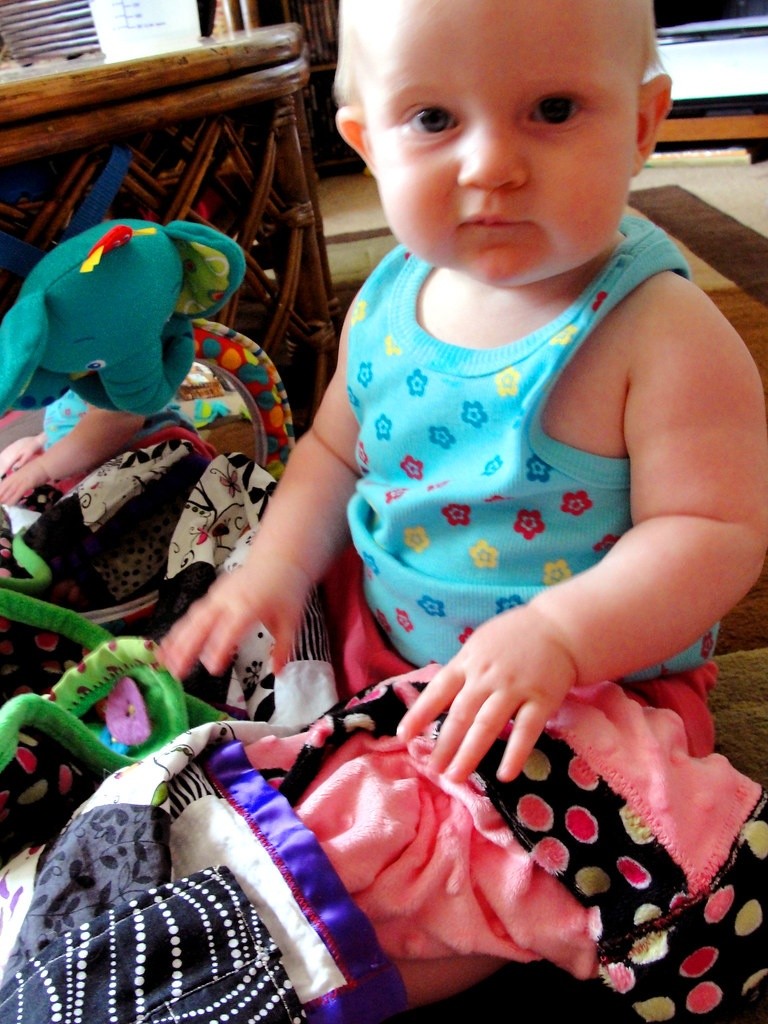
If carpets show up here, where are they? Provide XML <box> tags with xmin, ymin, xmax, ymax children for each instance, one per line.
<box><xmin>325</xmin><ymin>184</ymin><xmax>768</xmax><ymax>796</ymax></box>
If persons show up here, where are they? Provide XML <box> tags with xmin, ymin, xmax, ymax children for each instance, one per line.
<box><xmin>157</xmin><ymin>0</ymin><xmax>768</xmax><ymax>783</ymax></box>
<box><xmin>0</xmin><ymin>389</ymin><xmax>205</xmax><ymax>505</ymax></box>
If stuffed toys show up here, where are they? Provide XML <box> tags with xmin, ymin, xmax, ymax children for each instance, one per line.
<box><xmin>0</xmin><ymin>218</ymin><xmax>246</xmax><ymax>416</ymax></box>
